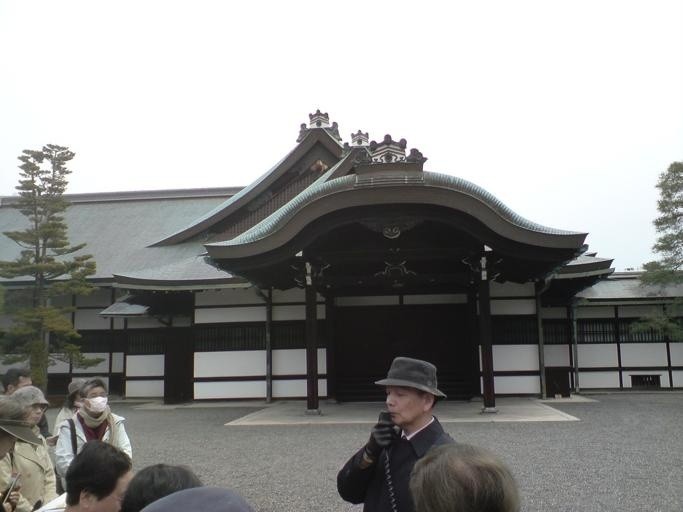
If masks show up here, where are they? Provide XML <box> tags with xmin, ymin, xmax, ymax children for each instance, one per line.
<box><xmin>80</xmin><ymin>396</ymin><xmax>108</xmax><ymax>412</ymax></box>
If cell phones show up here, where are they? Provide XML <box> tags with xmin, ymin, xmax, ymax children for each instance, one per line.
<box><xmin>3</xmin><ymin>473</ymin><xmax>23</xmax><ymax>505</ymax></box>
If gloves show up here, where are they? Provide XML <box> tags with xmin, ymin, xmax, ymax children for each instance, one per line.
<box><xmin>364</xmin><ymin>418</ymin><xmax>395</xmax><ymax>459</ymax></box>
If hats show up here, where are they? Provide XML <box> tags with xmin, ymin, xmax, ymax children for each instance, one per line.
<box><xmin>9</xmin><ymin>385</ymin><xmax>50</xmax><ymax>413</ymax></box>
<box><xmin>141</xmin><ymin>486</ymin><xmax>255</xmax><ymax>512</ymax></box>
<box><xmin>374</xmin><ymin>356</ymin><xmax>448</xmax><ymax>399</ymax></box>
<box><xmin>0</xmin><ymin>419</ymin><xmax>43</xmax><ymax>446</ymax></box>
<box><xmin>67</xmin><ymin>378</ymin><xmax>87</xmax><ymax>398</ymax></box>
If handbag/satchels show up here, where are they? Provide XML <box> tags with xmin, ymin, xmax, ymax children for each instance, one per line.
<box><xmin>55</xmin><ymin>466</ymin><xmax>66</xmax><ymax>495</ymax></box>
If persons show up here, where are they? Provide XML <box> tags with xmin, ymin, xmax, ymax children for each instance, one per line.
<box><xmin>53</xmin><ymin>376</ymin><xmax>134</xmax><ymax>491</ymax></box>
<box><xmin>406</xmin><ymin>439</ymin><xmax>520</xmax><ymax>511</ymax></box>
<box><xmin>114</xmin><ymin>461</ymin><xmax>205</xmax><ymax>511</ymax></box>
<box><xmin>51</xmin><ymin>377</ymin><xmax>88</xmax><ymax>476</ymax></box>
<box><xmin>2</xmin><ymin>366</ymin><xmax>51</xmax><ymax>439</ymax></box>
<box><xmin>59</xmin><ymin>440</ymin><xmax>136</xmax><ymax>511</ymax></box>
<box><xmin>0</xmin><ymin>397</ymin><xmax>42</xmax><ymax>512</ymax></box>
<box><xmin>0</xmin><ymin>384</ymin><xmax>57</xmax><ymax>512</ymax></box>
<box><xmin>333</xmin><ymin>354</ymin><xmax>457</xmax><ymax>512</ymax></box>
